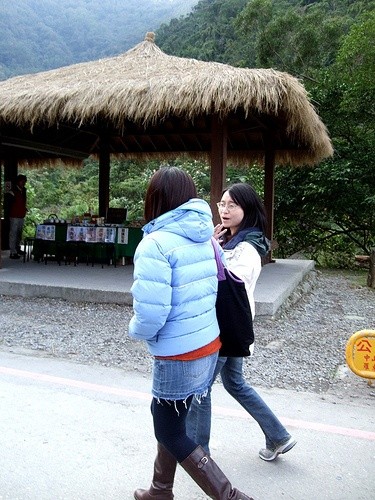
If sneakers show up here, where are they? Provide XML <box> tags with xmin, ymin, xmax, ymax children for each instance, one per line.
<box><xmin>258</xmin><ymin>436</ymin><xmax>298</xmax><ymax>461</ymax></box>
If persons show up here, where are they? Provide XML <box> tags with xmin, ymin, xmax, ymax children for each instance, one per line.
<box><xmin>4</xmin><ymin>174</ymin><xmax>27</xmax><ymax>259</ymax></box>
<box><xmin>212</xmin><ymin>184</ymin><xmax>297</xmax><ymax>461</ymax></box>
<box><xmin>128</xmin><ymin>166</ymin><xmax>254</xmax><ymax>500</ymax></box>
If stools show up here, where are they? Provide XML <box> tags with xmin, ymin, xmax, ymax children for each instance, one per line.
<box><xmin>24</xmin><ymin>237</ymin><xmax>37</xmax><ymax>263</ymax></box>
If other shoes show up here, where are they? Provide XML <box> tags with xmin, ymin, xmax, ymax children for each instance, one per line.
<box><xmin>17</xmin><ymin>250</ymin><xmax>26</xmax><ymax>255</ymax></box>
<box><xmin>9</xmin><ymin>252</ymin><xmax>20</xmax><ymax>259</ymax></box>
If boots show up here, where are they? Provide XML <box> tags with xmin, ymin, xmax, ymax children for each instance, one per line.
<box><xmin>179</xmin><ymin>443</ymin><xmax>255</xmax><ymax>500</ymax></box>
<box><xmin>134</xmin><ymin>441</ymin><xmax>176</xmax><ymax>500</ymax></box>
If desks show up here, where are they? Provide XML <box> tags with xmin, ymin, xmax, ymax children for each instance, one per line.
<box><xmin>39</xmin><ymin>222</ymin><xmax>144</xmax><ymax>266</ymax></box>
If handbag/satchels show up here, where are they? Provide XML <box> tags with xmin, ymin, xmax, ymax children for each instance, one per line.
<box><xmin>210</xmin><ymin>239</ymin><xmax>255</xmax><ymax>358</ymax></box>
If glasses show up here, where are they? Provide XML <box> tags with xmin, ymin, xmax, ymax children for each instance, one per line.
<box><xmin>216</xmin><ymin>202</ymin><xmax>239</xmax><ymax>209</ymax></box>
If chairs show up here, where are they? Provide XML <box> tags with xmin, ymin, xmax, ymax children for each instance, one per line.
<box><xmin>42</xmin><ymin>228</ymin><xmax>117</xmax><ymax>269</ymax></box>
